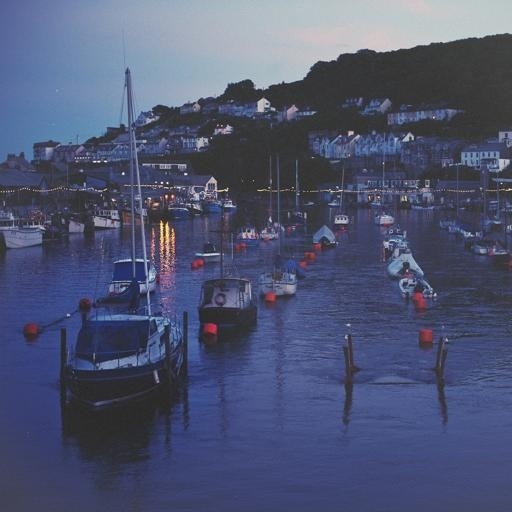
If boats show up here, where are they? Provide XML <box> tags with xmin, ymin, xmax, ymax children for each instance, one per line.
<box><xmin>108</xmin><ymin>257</ymin><xmax>158</xmax><ymax>295</ymax></box>
<box><xmin>195</xmin><ymin>243</ymin><xmax>225</xmax><ymax>262</ymax></box>
<box><xmin>368</xmin><ymin>197</ymin><xmax>436</xmax><ymax>299</ymax></box>
<box><xmin>198</xmin><ymin>275</ymin><xmax>258</xmax><ymax>328</ymax></box>
<box><xmin>313</xmin><ymin>226</ymin><xmax>336</xmax><ymax>246</ymax></box>
<box><xmin>260</xmin><ymin>266</ymin><xmax>299</xmax><ymax>296</ymax></box>
<box><xmin>2</xmin><ymin>189</ymin><xmax>237</xmax><ymax>249</ymax></box>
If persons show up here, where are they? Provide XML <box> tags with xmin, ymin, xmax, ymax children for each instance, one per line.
<box><xmin>126</xmin><ymin>278</ymin><xmax>140</xmax><ymax>314</ymax></box>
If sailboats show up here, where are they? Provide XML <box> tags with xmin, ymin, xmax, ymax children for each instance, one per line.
<box><xmin>238</xmin><ymin>156</ymin><xmax>306</xmax><ymax>247</ymax></box>
<box><xmin>439</xmin><ymin>162</ymin><xmax>512</xmax><ymax>256</ymax></box>
<box><xmin>65</xmin><ymin>67</ymin><xmax>182</xmax><ymax>420</ymax></box>
<box><xmin>328</xmin><ymin>167</ymin><xmax>350</xmax><ymax>231</ymax></box>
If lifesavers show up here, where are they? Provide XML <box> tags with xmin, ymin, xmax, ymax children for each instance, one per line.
<box><xmin>215</xmin><ymin>293</ymin><xmax>227</xmax><ymax>306</ymax></box>
